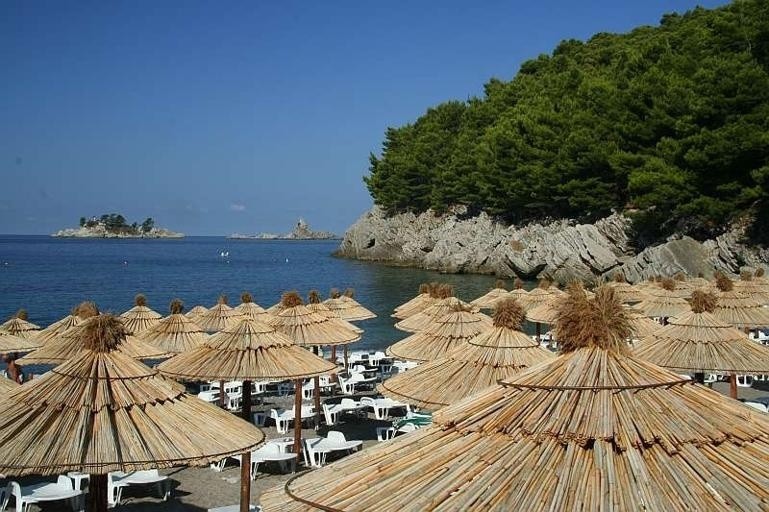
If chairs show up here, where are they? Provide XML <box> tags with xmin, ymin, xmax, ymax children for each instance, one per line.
<box><xmin>1</xmin><ymin>465</ymin><xmax>176</xmax><ymax>512</ymax></box>
<box><xmin>194</xmin><ymin>350</ymin><xmax>423</xmax><ymax>484</ymax></box>
